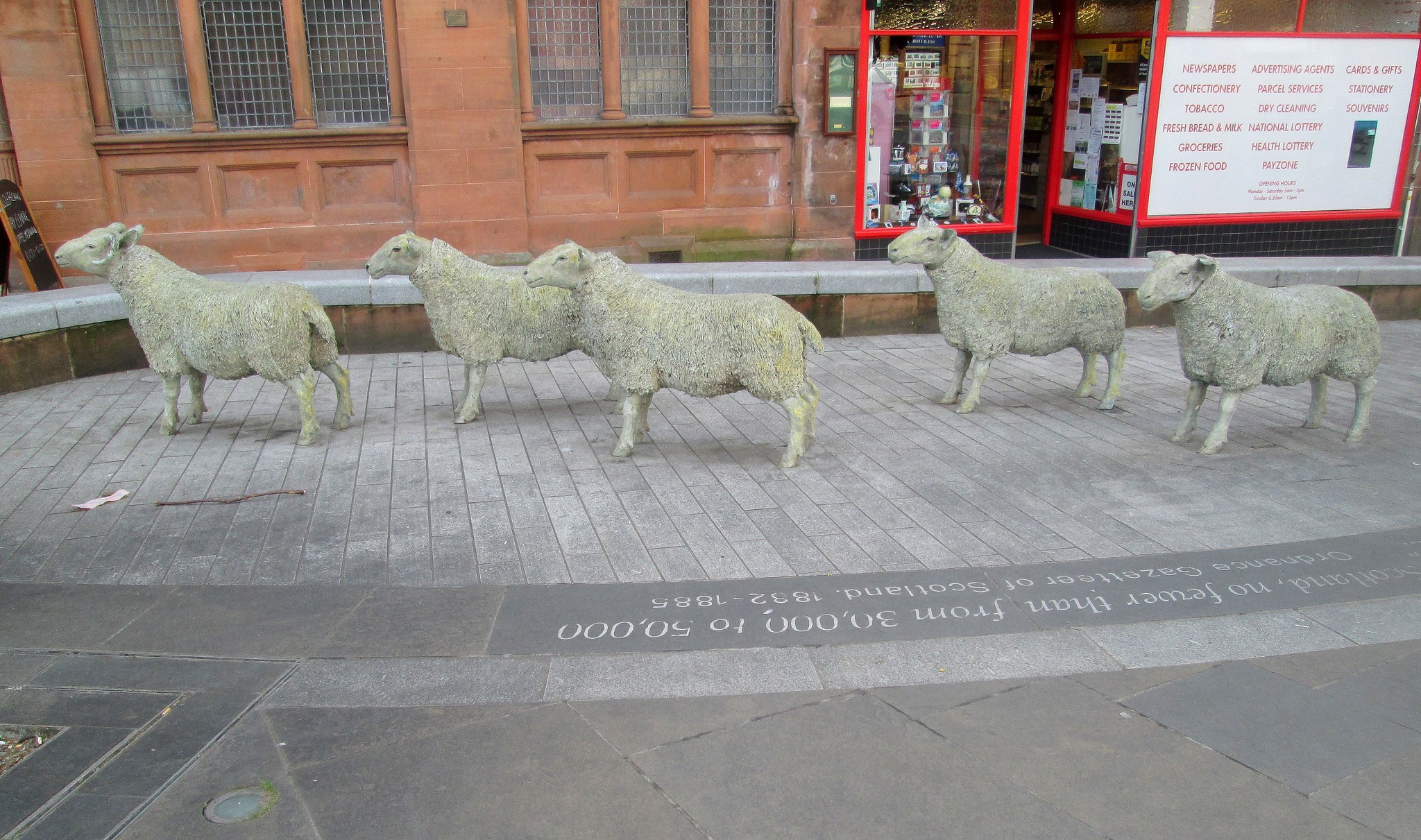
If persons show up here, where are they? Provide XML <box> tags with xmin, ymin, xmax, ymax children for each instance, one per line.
<box><xmin>972</xmin><ymin>207</ymin><xmax>979</xmax><ymax>213</ymax></box>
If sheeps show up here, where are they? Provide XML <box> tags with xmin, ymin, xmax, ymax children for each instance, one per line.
<box><xmin>54</xmin><ymin>220</ymin><xmax>355</xmax><ymax>445</ymax></box>
<box><xmin>521</xmin><ymin>237</ymin><xmax>827</xmax><ymax>468</ymax></box>
<box><xmin>364</xmin><ymin>227</ymin><xmax>629</xmax><ymax>425</ymax></box>
<box><xmin>884</xmin><ymin>213</ymin><xmax>1129</xmax><ymax>415</ymax></box>
<box><xmin>1135</xmin><ymin>246</ymin><xmax>1384</xmax><ymax>455</ymax></box>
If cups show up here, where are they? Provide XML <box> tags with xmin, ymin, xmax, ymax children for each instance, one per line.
<box><xmin>892</xmin><ymin>144</ymin><xmax>905</xmax><ymax>161</ymax></box>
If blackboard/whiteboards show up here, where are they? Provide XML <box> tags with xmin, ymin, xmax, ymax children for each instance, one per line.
<box><xmin>1</xmin><ymin>178</ymin><xmax>60</xmax><ymax>292</ymax></box>
